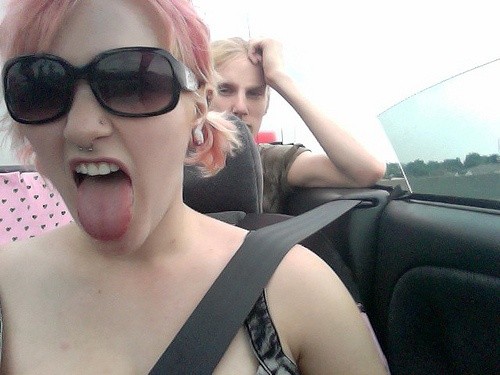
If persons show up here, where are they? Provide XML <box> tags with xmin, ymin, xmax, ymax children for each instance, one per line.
<box><xmin>199</xmin><ymin>37</ymin><xmax>387</xmax><ymax>219</ymax></box>
<box><xmin>1</xmin><ymin>0</ymin><xmax>391</xmax><ymax>374</ymax></box>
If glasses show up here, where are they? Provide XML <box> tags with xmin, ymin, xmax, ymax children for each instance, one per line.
<box><xmin>3</xmin><ymin>46</ymin><xmax>200</xmax><ymax>125</ymax></box>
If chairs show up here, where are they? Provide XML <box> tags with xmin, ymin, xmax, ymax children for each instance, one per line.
<box><xmin>183</xmin><ymin>115</ymin><xmax>362</xmax><ymax>316</ymax></box>
<box><xmin>280</xmin><ymin>181</ymin><xmax>500</xmax><ymax>375</ymax></box>
